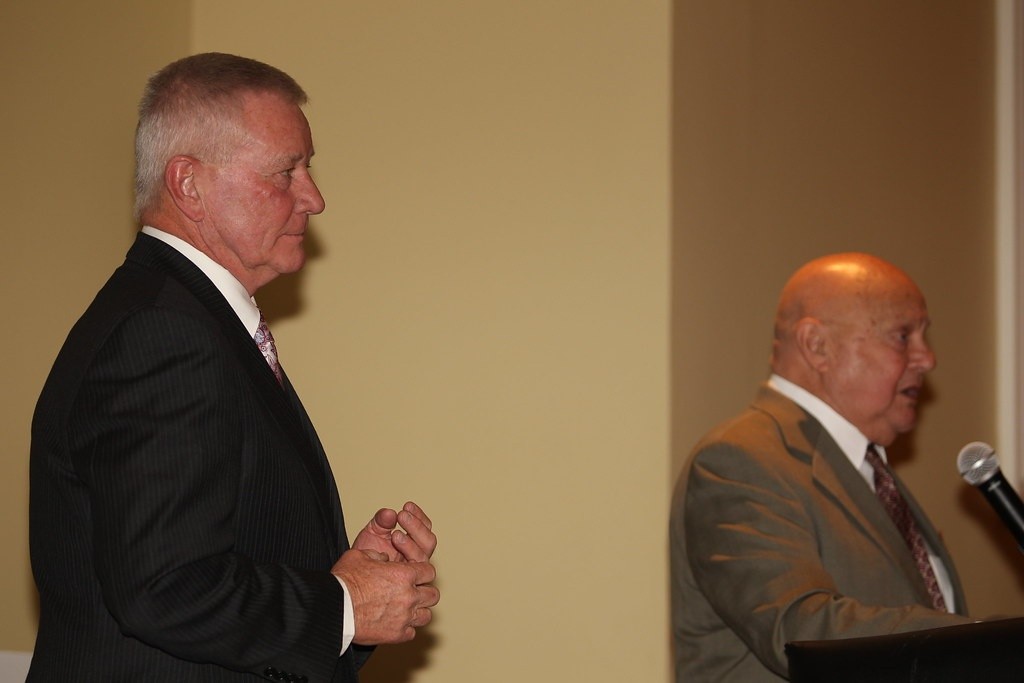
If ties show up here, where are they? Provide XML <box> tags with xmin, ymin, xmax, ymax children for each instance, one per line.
<box><xmin>253</xmin><ymin>306</ymin><xmax>284</xmax><ymax>385</ymax></box>
<box><xmin>865</xmin><ymin>444</ymin><xmax>948</xmax><ymax>614</ymax></box>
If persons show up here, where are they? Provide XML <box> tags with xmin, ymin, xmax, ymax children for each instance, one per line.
<box><xmin>24</xmin><ymin>51</ymin><xmax>440</xmax><ymax>683</ymax></box>
<box><xmin>669</xmin><ymin>252</ymin><xmax>1024</xmax><ymax>683</ymax></box>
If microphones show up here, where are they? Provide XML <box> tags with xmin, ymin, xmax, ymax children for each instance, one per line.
<box><xmin>957</xmin><ymin>441</ymin><xmax>1024</xmax><ymax>550</ymax></box>
